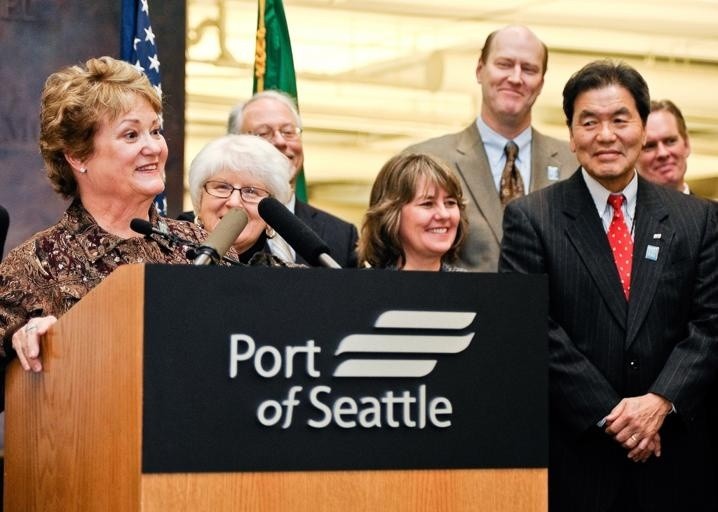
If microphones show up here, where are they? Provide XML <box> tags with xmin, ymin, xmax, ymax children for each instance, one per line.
<box><xmin>190</xmin><ymin>207</ymin><xmax>248</xmax><ymax>265</ymax></box>
<box><xmin>257</xmin><ymin>197</ymin><xmax>343</xmax><ymax>269</ymax></box>
<box><xmin>129</xmin><ymin>217</ymin><xmax>244</xmax><ymax>265</ymax></box>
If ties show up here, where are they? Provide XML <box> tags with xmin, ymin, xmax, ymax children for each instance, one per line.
<box><xmin>604</xmin><ymin>195</ymin><xmax>634</xmax><ymax>303</ymax></box>
<box><xmin>499</xmin><ymin>141</ymin><xmax>525</xmax><ymax>210</ymax></box>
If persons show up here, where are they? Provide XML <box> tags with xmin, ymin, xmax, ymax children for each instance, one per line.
<box><xmin>352</xmin><ymin>148</ymin><xmax>476</xmax><ymax>275</ymax></box>
<box><xmin>629</xmin><ymin>96</ymin><xmax>696</xmax><ymax>195</ymax></box>
<box><xmin>179</xmin><ymin>129</ymin><xmax>309</xmax><ymax>269</ymax></box>
<box><xmin>0</xmin><ymin>54</ymin><xmax>238</xmax><ymax>379</ymax></box>
<box><xmin>167</xmin><ymin>85</ymin><xmax>366</xmax><ymax>269</ymax></box>
<box><xmin>399</xmin><ymin>19</ymin><xmax>578</xmax><ymax>274</ymax></box>
<box><xmin>492</xmin><ymin>59</ymin><xmax>714</xmax><ymax>511</ymax></box>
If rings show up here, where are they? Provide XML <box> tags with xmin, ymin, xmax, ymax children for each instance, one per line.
<box><xmin>24</xmin><ymin>322</ymin><xmax>39</xmax><ymax>332</ymax></box>
<box><xmin>630</xmin><ymin>435</ymin><xmax>640</xmax><ymax>443</ymax></box>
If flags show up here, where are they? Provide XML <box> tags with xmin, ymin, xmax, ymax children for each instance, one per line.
<box><xmin>117</xmin><ymin>0</ymin><xmax>169</xmax><ymax>218</ymax></box>
<box><xmin>250</xmin><ymin>0</ymin><xmax>307</xmax><ymax>208</ymax></box>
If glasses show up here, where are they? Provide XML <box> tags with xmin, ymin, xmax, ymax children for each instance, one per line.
<box><xmin>247</xmin><ymin>123</ymin><xmax>303</xmax><ymax>141</ymax></box>
<box><xmin>200</xmin><ymin>181</ymin><xmax>273</xmax><ymax>205</ymax></box>
